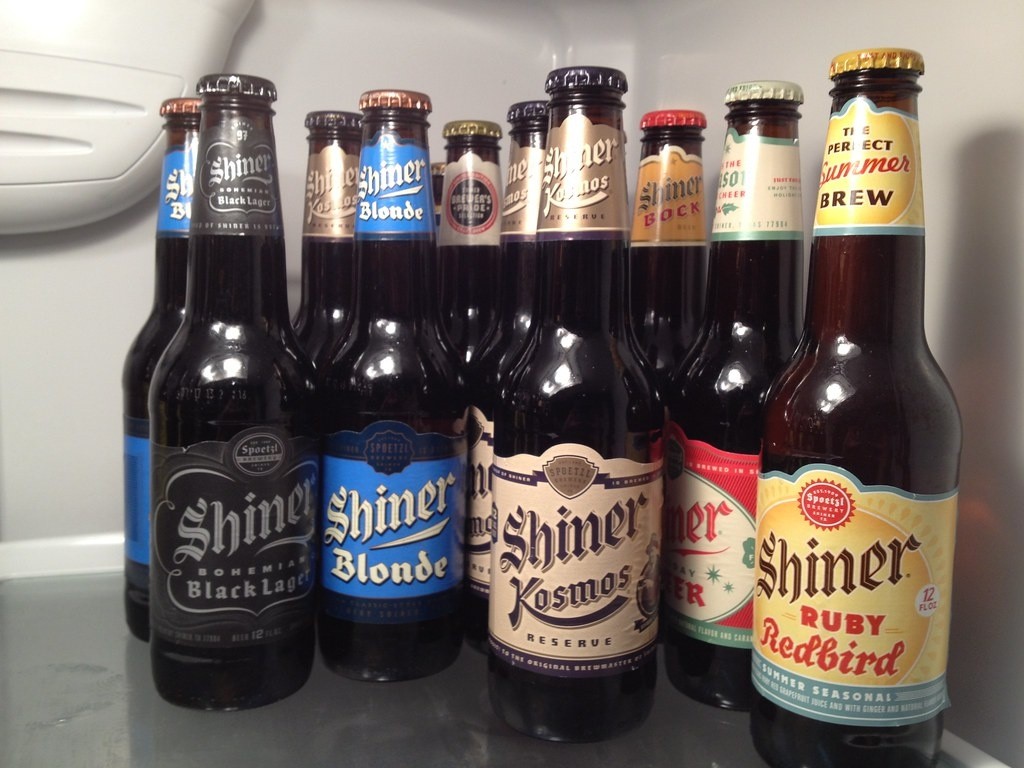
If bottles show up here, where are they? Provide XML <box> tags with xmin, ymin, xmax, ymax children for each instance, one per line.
<box><xmin>122</xmin><ymin>45</ymin><xmax>964</xmax><ymax>767</ymax></box>
